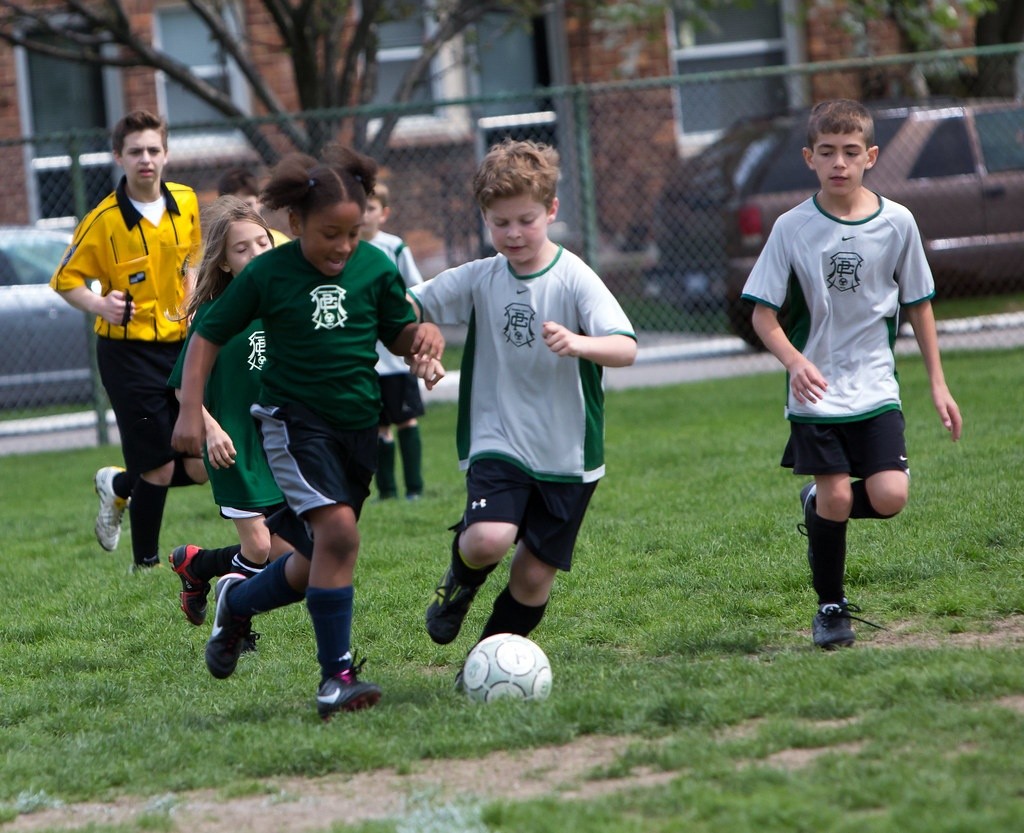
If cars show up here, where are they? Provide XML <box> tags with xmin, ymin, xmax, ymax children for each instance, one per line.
<box><xmin>0</xmin><ymin>227</ymin><xmax>99</xmax><ymax>408</ymax></box>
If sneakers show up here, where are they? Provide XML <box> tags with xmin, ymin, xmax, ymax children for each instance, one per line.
<box><xmin>426</xmin><ymin>564</ymin><xmax>480</xmax><ymax>645</ymax></box>
<box><xmin>130</xmin><ymin>564</ymin><xmax>164</xmax><ymax>579</ymax></box>
<box><xmin>317</xmin><ymin>649</ymin><xmax>382</xmax><ymax>723</ymax></box>
<box><xmin>242</xmin><ymin>629</ymin><xmax>259</xmax><ymax>654</ymax></box>
<box><xmin>811</xmin><ymin>598</ymin><xmax>888</xmax><ymax>648</ymax></box>
<box><xmin>169</xmin><ymin>544</ymin><xmax>212</xmax><ymax>626</ymax></box>
<box><xmin>94</xmin><ymin>466</ymin><xmax>129</xmax><ymax>553</ymax></box>
<box><xmin>205</xmin><ymin>572</ymin><xmax>251</xmax><ymax>680</ymax></box>
<box><xmin>797</xmin><ymin>484</ymin><xmax>824</xmax><ymax>566</ymax></box>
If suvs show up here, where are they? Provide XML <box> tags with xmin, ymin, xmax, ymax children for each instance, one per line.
<box><xmin>642</xmin><ymin>96</ymin><xmax>1024</xmax><ymax>351</ymax></box>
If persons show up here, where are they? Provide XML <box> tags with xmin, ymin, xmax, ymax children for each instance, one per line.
<box><xmin>404</xmin><ymin>141</ymin><xmax>638</xmax><ymax>695</ymax></box>
<box><xmin>357</xmin><ymin>185</ymin><xmax>426</xmax><ymax>501</ymax></box>
<box><xmin>741</xmin><ymin>97</ymin><xmax>963</xmax><ymax>650</ymax></box>
<box><xmin>217</xmin><ymin>171</ymin><xmax>292</xmax><ymax>247</ymax></box>
<box><xmin>168</xmin><ymin>202</ymin><xmax>297</xmax><ymax>655</ymax></box>
<box><xmin>49</xmin><ymin>107</ymin><xmax>208</xmax><ymax>579</ymax></box>
<box><xmin>171</xmin><ymin>147</ymin><xmax>445</xmax><ymax>724</ymax></box>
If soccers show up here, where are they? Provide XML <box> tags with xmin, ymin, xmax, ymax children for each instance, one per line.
<box><xmin>461</xmin><ymin>633</ymin><xmax>553</xmax><ymax>710</ymax></box>
